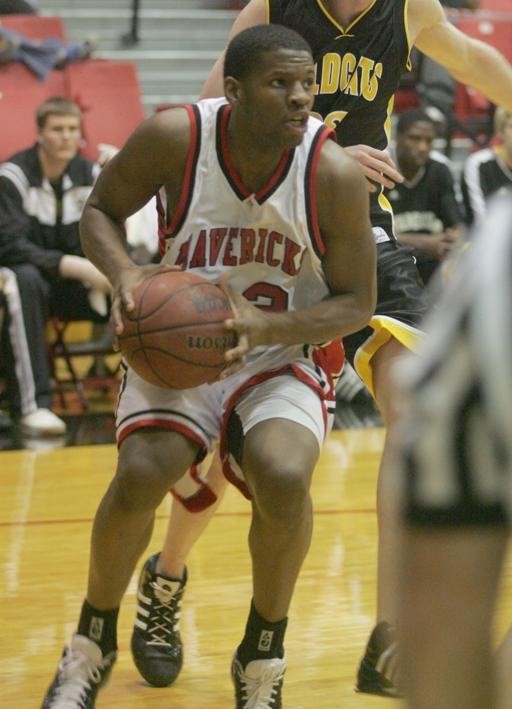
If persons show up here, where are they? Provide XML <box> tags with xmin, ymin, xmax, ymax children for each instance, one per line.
<box><xmin>42</xmin><ymin>1</ymin><xmax>512</xmax><ymax>709</ymax></box>
<box><xmin>0</xmin><ymin>99</ymin><xmax>113</xmax><ymax>434</ymax></box>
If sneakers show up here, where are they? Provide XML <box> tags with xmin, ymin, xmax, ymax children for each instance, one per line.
<box><xmin>15</xmin><ymin>407</ymin><xmax>66</xmax><ymax>437</ymax></box>
<box><xmin>131</xmin><ymin>552</ymin><xmax>187</xmax><ymax>686</ymax></box>
<box><xmin>229</xmin><ymin>624</ymin><xmax>287</xmax><ymax>709</ymax></box>
<box><xmin>40</xmin><ymin>627</ymin><xmax>117</xmax><ymax>708</ymax></box>
<box><xmin>357</xmin><ymin>620</ymin><xmax>407</xmax><ymax>699</ymax></box>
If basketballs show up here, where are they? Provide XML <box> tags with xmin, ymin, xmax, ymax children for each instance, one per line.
<box><xmin>117</xmin><ymin>272</ymin><xmax>235</xmax><ymax>390</ymax></box>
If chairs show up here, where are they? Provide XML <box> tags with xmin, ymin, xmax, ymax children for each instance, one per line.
<box><xmin>1</xmin><ymin>15</ymin><xmax>149</xmax><ymax>173</ymax></box>
